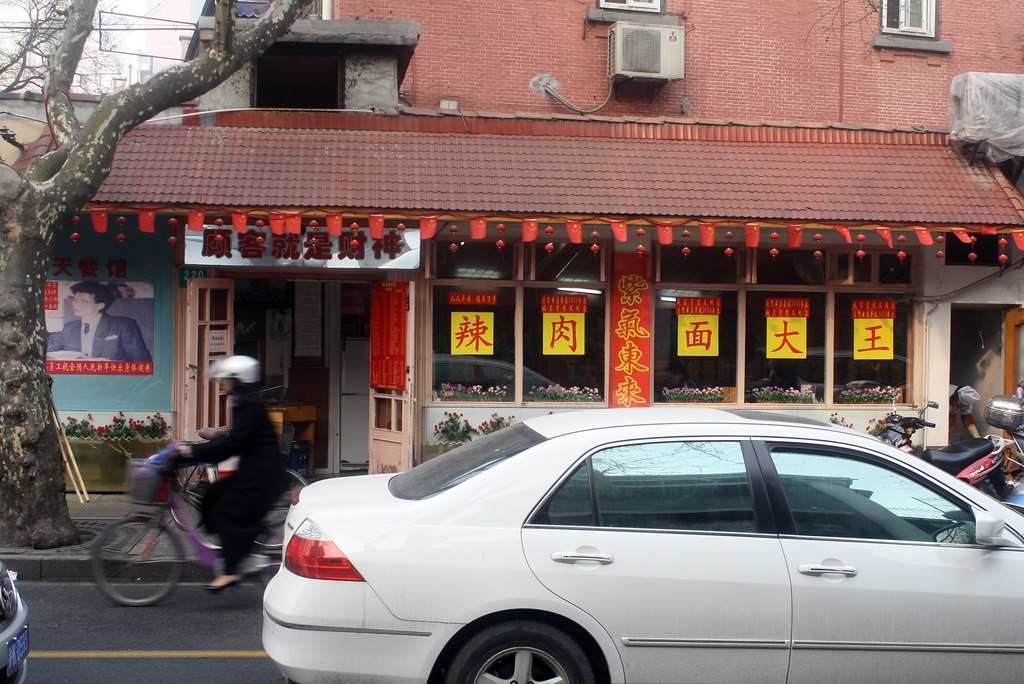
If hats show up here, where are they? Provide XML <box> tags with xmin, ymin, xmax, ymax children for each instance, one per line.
<box><xmin>958</xmin><ymin>386</ymin><xmax>982</xmax><ymax>406</ymax></box>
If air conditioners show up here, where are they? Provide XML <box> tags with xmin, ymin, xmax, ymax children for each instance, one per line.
<box><xmin>607</xmin><ymin>21</ymin><xmax>685</xmax><ymax>81</ymax></box>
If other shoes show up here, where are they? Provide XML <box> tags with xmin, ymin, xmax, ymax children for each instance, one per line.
<box><xmin>202</xmin><ymin>574</ymin><xmax>242</xmax><ymax>591</ymax></box>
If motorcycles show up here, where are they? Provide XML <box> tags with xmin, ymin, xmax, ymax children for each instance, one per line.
<box><xmin>869</xmin><ymin>394</ymin><xmax>1024</xmax><ymax>500</ymax></box>
<box><xmin>1000</xmin><ymin>425</ymin><xmax>1024</xmax><ymax>515</ymax></box>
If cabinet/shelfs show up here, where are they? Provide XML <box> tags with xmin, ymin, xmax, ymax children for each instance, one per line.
<box><xmin>265</xmin><ymin>405</ymin><xmax>316</xmax><ymax>477</ymax></box>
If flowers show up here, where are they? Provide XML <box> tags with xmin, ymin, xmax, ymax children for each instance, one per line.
<box><xmin>434</xmin><ymin>411</ymin><xmax>515</xmax><ymax>442</ymax></box>
<box><xmin>838</xmin><ymin>384</ymin><xmax>901</xmax><ymax>403</ymax></box>
<box><xmin>752</xmin><ymin>385</ymin><xmax>816</xmax><ymax>403</ymax></box>
<box><xmin>830</xmin><ymin>412</ymin><xmax>893</xmax><ymax>436</ymax></box>
<box><xmin>61</xmin><ymin>410</ymin><xmax>171</xmax><ymax>436</ymax></box>
<box><xmin>530</xmin><ymin>383</ymin><xmax>602</xmax><ymax>402</ymax></box>
<box><xmin>662</xmin><ymin>387</ymin><xmax>723</xmax><ymax>402</ymax></box>
<box><xmin>441</xmin><ymin>383</ymin><xmax>507</xmax><ymax>401</ymax></box>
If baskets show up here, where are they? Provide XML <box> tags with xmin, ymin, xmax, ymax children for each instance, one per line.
<box><xmin>125</xmin><ymin>458</ymin><xmax>159</xmax><ymax>500</ymax></box>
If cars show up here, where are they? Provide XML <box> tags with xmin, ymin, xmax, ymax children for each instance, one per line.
<box><xmin>260</xmin><ymin>406</ymin><xmax>1024</xmax><ymax>684</ymax></box>
<box><xmin>0</xmin><ymin>559</ymin><xmax>31</xmax><ymax>684</ymax></box>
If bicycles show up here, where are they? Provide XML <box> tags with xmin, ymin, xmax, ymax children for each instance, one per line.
<box><xmin>167</xmin><ymin>427</ymin><xmax>308</xmax><ymax>547</ymax></box>
<box><xmin>88</xmin><ymin>437</ymin><xmax>293</xmax><ymax>606</ymax></box>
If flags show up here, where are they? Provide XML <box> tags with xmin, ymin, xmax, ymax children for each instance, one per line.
<box><xmin>521</xmin><ymin>219</ymin><xmax>1024</xmax><ymax>251</ymax></box>
<box><xmin>90</xmin><ymin>208</ymin><xmax>487</xmax><ymax>240</ymax></box>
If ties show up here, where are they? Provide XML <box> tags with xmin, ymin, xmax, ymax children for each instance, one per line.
<box><xmin>84</xmin><ymin>323</ymin><xmax>89</xmax><ymax>334</ymax></box>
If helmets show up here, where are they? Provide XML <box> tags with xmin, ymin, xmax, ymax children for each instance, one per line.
<box><xmin>210</xmin><ymin>355</ymin><xmax>261</xmax><ymax>392</ymax></box>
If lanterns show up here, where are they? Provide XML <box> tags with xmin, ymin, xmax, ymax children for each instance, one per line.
<box><xmin>215</xmin><ymin>219</ymin><xmax>224</xmax><ymax>243</ymax></box>
<box><xmin>310</xmin><ymin>221</ymin><xmax>318</xmax><ymax>246</ymax></box>
<box><xmin>70</xmin><ymin>216</ymin><xmax>81</xmax><ymax>243</ymax></box>
<box><xmin>591</xmin><ymin>231</ymin><xmax>600</xmax><ymax>253</ymax></box>
<box><xmin>449</xmin><ymin>225</ymin><xmax>458</xmax><ymax>252</ymax></box>
<box><xmin>169</xmin><ymin>218</ymin><xmax>178</xmax><ymax>244</ymax></box>
<box><xmin>256</xmin><ymin>220</ymin><xmax>265</xmax><ymax>244</ymax></box>
<box><xmin>118</xmin><ymin>217</ymin><xmax>126</xmax><ymax>243</ymax></box>
<box><xmin>770</xmin><ymin>232</ymin><xmax>1007</xmax><ymax>262</ymax></box>
<box><xmin>396</xmin><ymin>224</ymin><xmax>405</xmax><ymax>247</ymax></box>
<box><xmin>636</xmin><ymin>228</ymin><xmax>646</xmax><ymax>253</ymax></box>
<box><xmin>545</xmin><ymin>225</ymin><xmax>555</xmax><ymax>253</ymax></box>
<box><xmin>681</xmin><ymin>231</ymin><xmax>691</xmax><ymax>255</ymax></box>
<box><xmin>724</xmin><ymin>231</ymin><xmax>734</xmax><ymax>256</ymax></box>
<box><xmin>496</xmin><ymin>224</ymin><xmax>505</xmax><ymax>248</ymax></box>
<box><xmin>350</xmin><ymin>222</ymin><xmax>359</xmax><ymax>247</ymax></box>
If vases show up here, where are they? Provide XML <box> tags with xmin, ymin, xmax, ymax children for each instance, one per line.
<box><xmin>62</xmin><ymin>437</ymin><xmax>170</xmax><ymax>491</ymax></box>
<box><xmin>840</xmin><ymin>399</ymin><xmax>899</xmax><ymax>403</ymax></box>
<box><xmin>533</xmin><ymin>396</ymin><xmax>601</xmax><ymax>402</ymax></box>
<box><xmin>665</xmin><ymin>398</ymin><xmax>721</xmax><ymax>403</ymax></box>
<box><xmin>755</xmin><ymin>397</ymin><xmax>813</xmax><ymax>404</ymax></box>
<box><xmin>422</xmin><ymin>441</ymin><xmax>462</xmax><ymax>462</ymax></box>
<box><xmin>441</xmin><ymin>395</ymin><xmax>503</xmax><ymax>402</ymax></box>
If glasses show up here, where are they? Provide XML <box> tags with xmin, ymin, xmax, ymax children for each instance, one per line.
<box><xmin>67</xmin><ymin>296</ymin><xmax>97</xmax><ymax>306</ymax></box>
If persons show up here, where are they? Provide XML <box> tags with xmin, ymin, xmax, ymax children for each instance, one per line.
<box><xmin>155</xmin><ymin>356</ymin><xmax>281</xmax><ymax>589</ymax></box>
<box><xmin>949</xmin><ymin>383</ymin><xmax>983</xmax><ymax>441</ymax></box>
<box><xmin>44</xmin><ymin>281</ymin><xmax>154</xmax><ymax>361</ymax></box>
